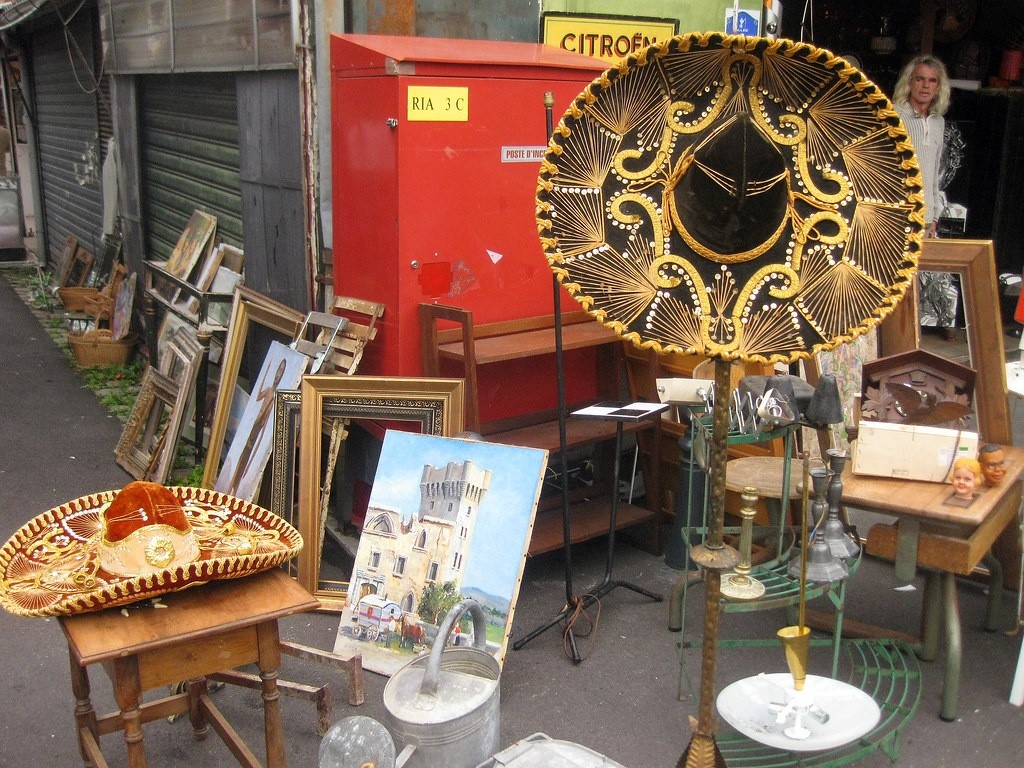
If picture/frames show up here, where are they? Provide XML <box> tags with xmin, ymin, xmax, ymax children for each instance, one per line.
<box><xmin>46</xmin><ymin>213</ymin><xmax>466</xmax><ymax>614</ymax></box>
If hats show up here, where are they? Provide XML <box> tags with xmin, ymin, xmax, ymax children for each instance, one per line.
<box><xmin>0</xmin><ymin>479</ymin><xmax>304</xmax><ymax>617</ymax></box>
<box><xmin>537</xmin><ymin>30</ymin><xmax>924</xmax><ymax>361</ymax></box>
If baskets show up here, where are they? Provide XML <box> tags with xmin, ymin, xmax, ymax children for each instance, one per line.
<box><xmin>71</xmin><ymin>309</ymin><xmax>138</xmax><ymax>368</ymax></box>
<box><xmin>84</xmin><ymin>291</ymin><xmax>113</xmax><ymax>319</ymax></box>
<box><xmin>58</xmin><ymin>287</ymin><xmax>98</xmax><ymax>309</ymax></box>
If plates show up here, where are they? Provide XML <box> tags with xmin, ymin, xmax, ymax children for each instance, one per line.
<box><xmin>714</xmin><ymin>672</ymin><xmax>882</xmax><ymax>753</ymax></box>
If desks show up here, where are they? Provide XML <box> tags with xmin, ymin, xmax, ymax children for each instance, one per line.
<box><xmin>970</xmin><ymin>86</ymin><xmax>1024</xmax><ymax>268</ymax></box>
<box><xmin>56</xmin><ymin>568</ymin><xmax>322</xmax><ymax>768</ymax></box>
<box><xmin>795</xmin><ymin>438</ymin><xmax>1024</xmax><ymax>723</ymax></box>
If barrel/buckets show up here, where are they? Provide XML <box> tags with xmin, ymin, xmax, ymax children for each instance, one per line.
<box><xmin>383</xmin><ymin>598</ymin><xmax>502</xmax><ymax>768</ymax></box>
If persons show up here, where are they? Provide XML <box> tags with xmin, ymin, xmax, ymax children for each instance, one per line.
<box><xmin>979</xmin><ymin>444</ymin><xmax>1008</xmax><ymax>488</ymax></box>
<box><xmin>948</xmin><ymin>454</ymin><xmax>981</xmax><ymax>501</ymax></box>
<box><xmin>892</xmin><ymin>54</ymin><xmax>957</xmax><ymax>341</ymax></box>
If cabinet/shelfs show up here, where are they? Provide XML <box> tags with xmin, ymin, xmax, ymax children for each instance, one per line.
<box><xmin>676</xmin><ymin>402</ymin><xmax>921</xmax><ymax>768</ymax></box>
<box><xmin>418</xmin><ymin>300</ymin><xmax>667</xmax><ymax>583</ymax></box>
